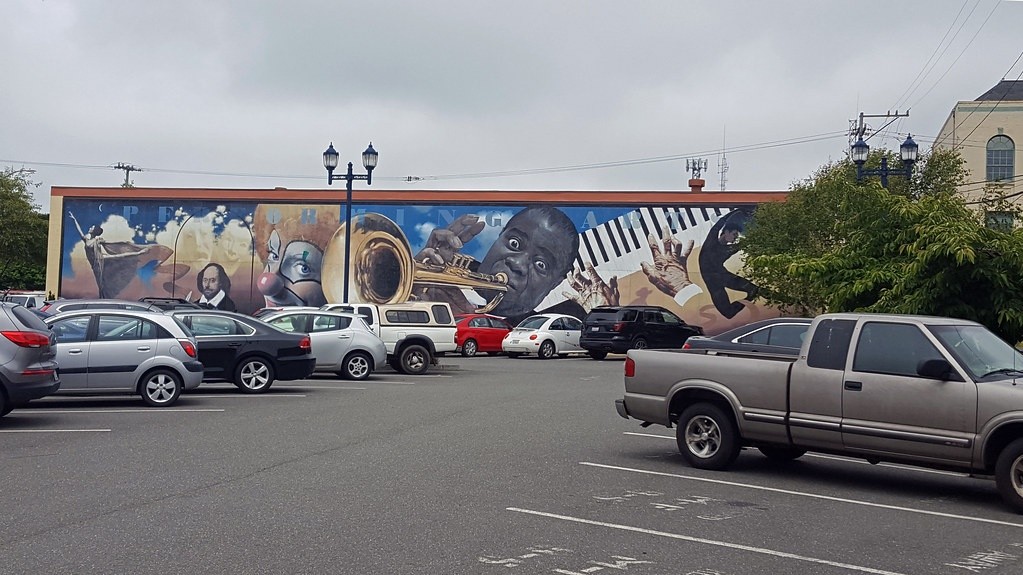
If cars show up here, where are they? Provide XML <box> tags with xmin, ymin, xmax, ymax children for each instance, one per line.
<box><xmin>0</xmin><ymin>295</ymin><xmax>388</xmax><ymax>418</ymax></box>
<box><xmin>166</xmin><ymin>310</ymin><xmax>316</xmax><ymax>394</ymax></box>
<box><xmin>501</xmin><ymin>313</ymin><xmax>589</xmax><ymax>360</ymax></box>
<box><xmin>452</xmin><ymin>313</ymin><xmax>514</xmax><ymax>357</ymax></box>
<box><xmin>41</xmin><ymin>309</ymin><xmax>204</xmax><ymax>408</ymax></box>
<box><xmin>680</xmin><ymin>316</ymin><xmax>813</xmax><ymax>363</ymax></box>
<box><xmin>579</xmin><ymin>305</ymin><xmax>704</xmax><ymax>361</ymax></box>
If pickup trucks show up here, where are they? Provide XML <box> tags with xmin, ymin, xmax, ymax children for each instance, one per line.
<box><xmin>313</xmin><ymin>302</ymin><xmax>457</xmax><ymax>376</ymax></box>
<box><xmin>614</xmin><ymin>313</ymin><xmax>1023</xmax><ymax>514</ymax></box>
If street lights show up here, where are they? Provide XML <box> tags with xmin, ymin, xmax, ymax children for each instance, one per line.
<box><xmin>322</xmin><ymin>141</ymin><xmax>379</xmax><ymax>303</ymax></box>
<box><xmin>851</xmin><ymin>133</ymin><xmax>919</xmax><ymax>190</ymax></box>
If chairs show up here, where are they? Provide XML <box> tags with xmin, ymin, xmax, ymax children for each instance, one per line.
<box><xmin>290</xmin><ymin>316</ymin><xmax>305</xmax><ymax>333</ymax></box>
